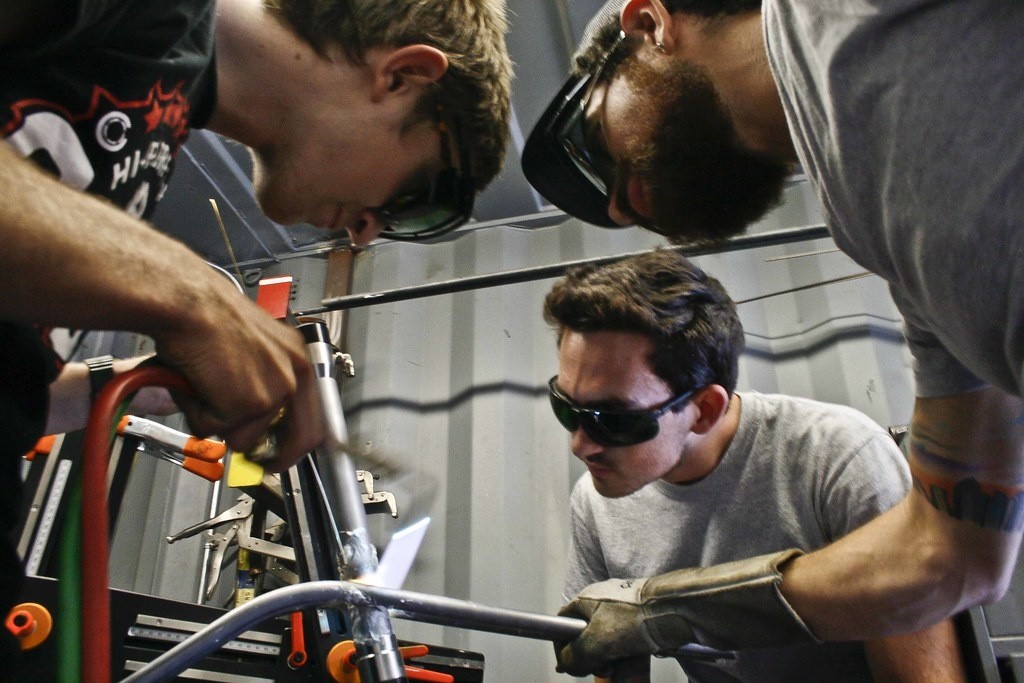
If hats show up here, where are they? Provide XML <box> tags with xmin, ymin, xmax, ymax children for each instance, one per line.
<box><xmin>518</xmin><ymin>0</ymin><xmax>641</xmax><ymax>232</ymax></box>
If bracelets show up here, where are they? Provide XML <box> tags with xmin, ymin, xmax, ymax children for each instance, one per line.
<box><xmin>84</xmin><ymin>354</ymin><xmax>115</xmax><ymax>410</ymax></box>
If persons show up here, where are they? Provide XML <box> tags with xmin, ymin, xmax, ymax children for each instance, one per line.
<box><xmin>520</xmin><ymin>0</ymin><xmax>1024</xmax><ymax>679</ymax></box>
<box><xmin>542</xmin><ymin>252</ymin><xmax>967</xmax><ymax>683</ymax></box>
<box><xmin>1</xmin><ymin>0</ymin><xmax>519</xmax><ymax>682</ymax></box>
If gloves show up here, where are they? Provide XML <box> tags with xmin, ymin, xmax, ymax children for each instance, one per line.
<box><xmin>556</xmin><ymin>546</ymin><xmax>823</xmax><ymax>675</ymax></box>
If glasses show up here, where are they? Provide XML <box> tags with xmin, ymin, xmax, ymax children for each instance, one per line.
<box><xmin>556</xmin><ymin>28</ymin><xmax>688</xmax><ymax>243</ymax></box>
<box><xmin>381</xmin><ymin>100</ymin><xmax>470</xmax><ymax>240</ymax></box>
<box><xmin>545</xmin><ymin>373</ymin><xmax>711</xmax><ymax>447</ymax></box>
